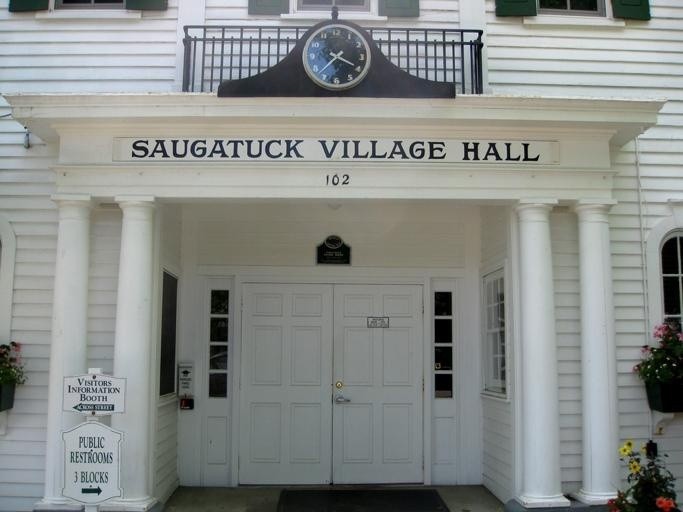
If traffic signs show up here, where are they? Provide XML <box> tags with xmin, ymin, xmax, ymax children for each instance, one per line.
<box><xmin>61</xmin><ymin>374</ymin><xmax>126</xmax><ymax>416</ymax></box>
<box><xmin>59</xmin><ymin>421</ymin><xmax>124</xmax><ymax>507</ymax></box>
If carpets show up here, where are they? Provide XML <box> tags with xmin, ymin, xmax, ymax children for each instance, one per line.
<box><xmin>273</xmin><ymin>487</ymin><xmax>450</xmax><ymax>512</ymax></box>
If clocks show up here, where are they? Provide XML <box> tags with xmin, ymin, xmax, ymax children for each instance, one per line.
<box><xmin>300</xmin><ymin>23</ymin><xmax>373</xmax><ymax>94</ymax></box>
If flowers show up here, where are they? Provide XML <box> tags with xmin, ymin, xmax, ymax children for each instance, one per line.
<box><xmin>632</xmin><ymin>316</ymin><xmax>683</xmax><ymax>377</ymax></box>
<box><xmin>605</xmin><ymin>433</ymin><xmax>683</xmax><ymax>512</ymax></box>
<box><xmin>0</xmin><ymin>341</ymin><xmax>34</xmax><ymax>382</ymax></box>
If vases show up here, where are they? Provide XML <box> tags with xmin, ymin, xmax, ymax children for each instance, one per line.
<box><xmin>0</xmin><ymin>376</ymin><xmax>19</xmax><ymax>411</ymax></box>
<box><xmin>643</xmin><ymin>371</ymin><xmax>683</xmax><ymax>414</ymax></box>
